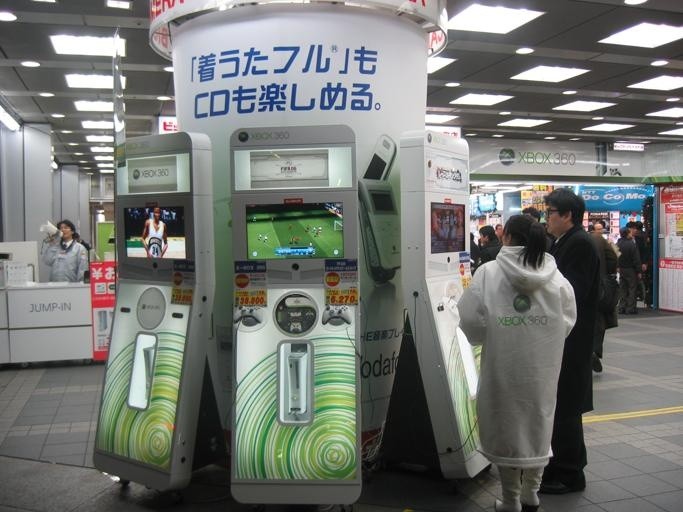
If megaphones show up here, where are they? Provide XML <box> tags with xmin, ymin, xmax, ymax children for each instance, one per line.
<box><xmin>41</xmin><ymin>220</ymin><xmax>65</xmax><ymax>243</ymax></box>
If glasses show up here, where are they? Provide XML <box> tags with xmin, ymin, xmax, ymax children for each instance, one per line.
<box><xmin>543</xmin><ymin>209</ymin><xmax>560</xmax><ymax>217</ymax></box>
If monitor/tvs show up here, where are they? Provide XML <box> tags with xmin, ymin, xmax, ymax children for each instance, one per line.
<box><xmin>430</xmin><ymin>202</ymin><xmax>465</xmax><ymax>254</ymax></box>
<box><xmin>245</xmin><ymin>202</ymin><xmax>345</xmax><ymax>260</ymax></box>
<box><xmin>124</xmin><ymin>205</ymin><xmax>186</xmax><ymax>259</ymax></box>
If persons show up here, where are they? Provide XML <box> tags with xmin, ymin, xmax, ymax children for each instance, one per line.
<box><xmin>457</xmin><ymin>187</ymin><xmax>618</xmax><ymax>511</ymax></box>
<box><xmin>615</xmin><ymin>221</ymin><xmax>654</xmax><ymax>313</ymax></box>
<box><xmin>73</xmin><ymin>232</ymin><xmax>90</xmax><ymax>249</ymax></box>
<box><xmin>41</xmin><ymin>219</ymin><xmax>90</xmax><ymax>282</ymax></box>
<box><xmin>140</xmin><ymin>208</ymin><xmax>168</xmax><ymax>258</ymax></box>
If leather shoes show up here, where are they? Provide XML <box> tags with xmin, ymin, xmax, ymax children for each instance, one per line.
<box><xmin>540</xmin><ymin>480</ymin><xmax>584</xmax><ymax>494</ymax></box>
<box><xmin>592</xmin><ymin>352</ymin><xmax>602</xmax><ymax>372</ymax></box>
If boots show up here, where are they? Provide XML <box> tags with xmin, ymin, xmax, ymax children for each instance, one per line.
<box><xmin>496</xmin><ymin>466</ymin><xmax>544</xmax><ymax>512</ymax></box>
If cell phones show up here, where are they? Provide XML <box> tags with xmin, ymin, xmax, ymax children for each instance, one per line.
<box><xmin>362</xmin><ymin>133</ymin><xmax>397</xmax><ymax>180</ymax></box>
<box><xmin>357</xmin><ymin>177</ymin><xmax>401</xmax><ymax>285</ymax></box>
